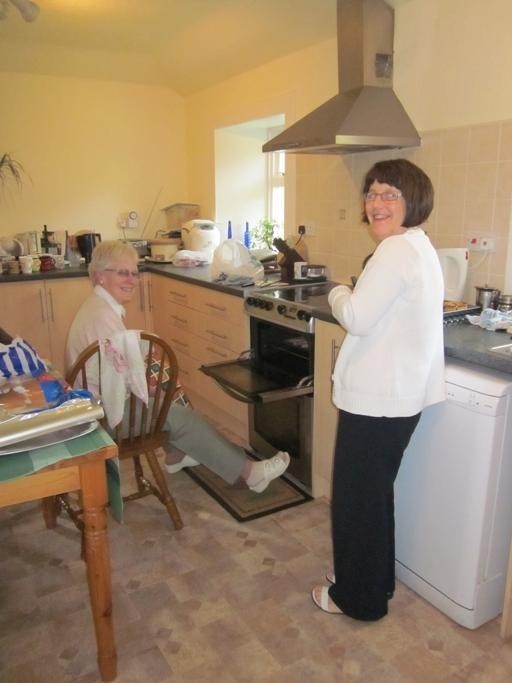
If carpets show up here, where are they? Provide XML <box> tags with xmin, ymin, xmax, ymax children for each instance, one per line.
<box><xmin>179</xmin><ymin>444</ymin><xmax>316</xmax><ymax>526</ymax></box>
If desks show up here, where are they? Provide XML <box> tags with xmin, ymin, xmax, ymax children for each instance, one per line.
<box><xmin>1</xmin><ymin>354</ymin><xmax>118</xmax><ymax>678</ymax></box>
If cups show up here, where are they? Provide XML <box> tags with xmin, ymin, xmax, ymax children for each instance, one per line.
<box><xmin>476</xmin><ymin>283</ymin><xmax>502</xmax><ymax>312</ymax></box>
<box><xmin>7</xmin><ymin>253</ymin><xmax>63</xmax><ymax>275</ymax></box>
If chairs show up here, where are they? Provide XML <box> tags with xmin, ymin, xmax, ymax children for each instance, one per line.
<box><xmin>47</xmin><ymin>329</ymin><xmax>184</xmax><ymax>560</ymax></box>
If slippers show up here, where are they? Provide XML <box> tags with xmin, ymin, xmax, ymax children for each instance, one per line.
<box><xmin>163</xmin><ymin>454</ymin><xmax>201</xmax><ymax>474</ymax></box>
<box><xmin>249</xmin><ymin>451</ymin><xmax>290</xmax><ymax>494</ymax></box>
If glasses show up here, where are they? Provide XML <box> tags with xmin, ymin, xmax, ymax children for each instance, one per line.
<box><xmin>107</xmin><ymin>266</ymin><xmax>139</xmax><ymax>277</ymax></box>
<box><xmin>363</xmin><ymin>190</ymin><xmax>402</xmax><ymax>201</ymax></box>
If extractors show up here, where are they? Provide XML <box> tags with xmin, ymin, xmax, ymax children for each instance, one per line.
<box><xmin>260</xmin><ymin>0</ymin><xmax>421</xmax><ymax>153</ymax></box>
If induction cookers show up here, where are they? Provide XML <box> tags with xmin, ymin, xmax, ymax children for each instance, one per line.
<box><xmin>243</xmin><ymin>281</ymin><xmax>361</xmax><ymax>332</ymax></box>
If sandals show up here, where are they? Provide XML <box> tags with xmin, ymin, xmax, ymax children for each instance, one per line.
<box><xmin>311</xmin><ymin>572</ymin><xmax>393</xmax><ymax>615</ymax></box>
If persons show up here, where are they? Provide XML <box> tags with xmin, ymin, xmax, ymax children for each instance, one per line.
<box><xmin>311</xmin><ymin>158</ymin><xmax>451</xmax><ymax>620</ymax></box>
<box><xmin>65</xmin><ymin>238</ymin><xmax>291</xmax><ymax>494</ymax></box>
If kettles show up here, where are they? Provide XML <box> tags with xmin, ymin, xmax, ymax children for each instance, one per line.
<box><xmin>77</xmin><ymin>230</ymin><xmax>102</xmax><ymax>264</ymax></box>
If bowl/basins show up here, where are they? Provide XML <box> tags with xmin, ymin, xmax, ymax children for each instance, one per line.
<box><xmin>302</xmin><ymin>264</ymin><xmax>325</xmax><ymax>278</ymax></box>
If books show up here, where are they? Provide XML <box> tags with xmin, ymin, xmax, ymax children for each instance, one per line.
<box><xmin>0</xmin><ymin>357</ymin><xmax>104</xmax><ymax>449</ymax></box>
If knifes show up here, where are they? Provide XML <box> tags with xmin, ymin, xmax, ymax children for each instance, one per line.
<box><xmin>272</xmin><ymin>237</ymin><xmax>291</xmax><ymax>255</ymax></box>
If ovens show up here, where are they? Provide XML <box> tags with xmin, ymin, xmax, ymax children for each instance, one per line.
<box><xmin>198</xmin><ymin>316</ymin><xmax>312</xmax><ymax>492</ymax></box>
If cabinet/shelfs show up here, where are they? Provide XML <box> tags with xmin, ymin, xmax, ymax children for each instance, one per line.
<box><xmin>148</xmin><ymin>273</ymin><xmax>250</xmax><ymax>448</ymax></box>
<box><xmin>308</xmin><ymin>317</ymin><xmax>352</xmax><ymax>513</ymax></box>
<box><xmin>0</xmin><ymin>271</ymin><xmax>139</xmax><ymax>376</ymax></box>
